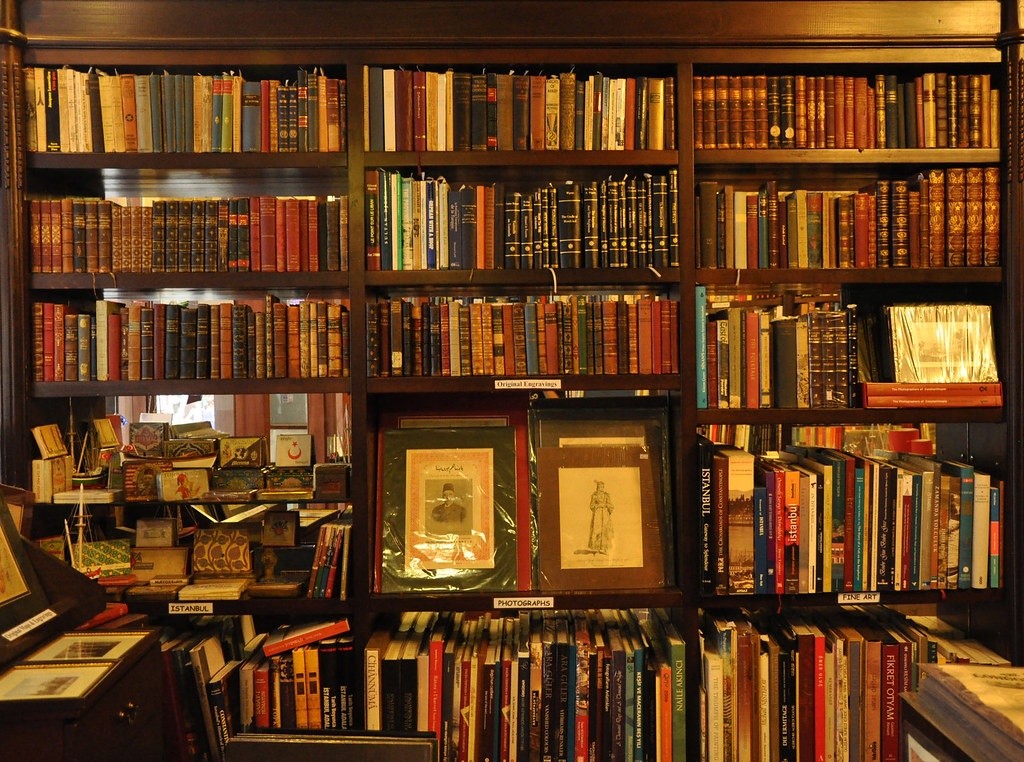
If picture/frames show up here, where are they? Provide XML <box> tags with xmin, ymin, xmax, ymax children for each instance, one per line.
<box><xmin>0</xmin><ymin>493</ymin><xmax>51</xmax><ymax>635</ymax></box>
<box><xmin>0</xmin><ymin>661</ymin><xmax>125</xmax><ymax>713</ymax></box>
<box><xmin>13</xmin><ymin>631</ymin><xmax>163</xmax><ymax>670</ymax></box>
<box><xmin>0</xmin><ymin>482</ymin><xmax>35</xmax><ymax>538</ymax></box>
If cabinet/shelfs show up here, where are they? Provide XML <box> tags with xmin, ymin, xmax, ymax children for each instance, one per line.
<box><xmin>13</xmin><ymin>47</ymin><xmax>1024</xmax><ymax>762</ymax></box>
<box><xmin>0</xmin><ymin>626</ymin><xmax>189</xmax><ymax>762</ymax></box>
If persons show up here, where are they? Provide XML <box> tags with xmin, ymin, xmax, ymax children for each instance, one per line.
<box><xmin>432</xmin><ymin>482</ymin><xmax>467</xmax><ymax>522</ymax></box>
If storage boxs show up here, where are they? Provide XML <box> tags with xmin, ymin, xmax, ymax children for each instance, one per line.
<box><xmin>32</xmin><ymin>416</ymin><xmax>350</xmax><ymax>603</ymax></box>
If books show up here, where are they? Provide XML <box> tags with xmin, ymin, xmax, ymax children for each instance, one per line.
<box><xmin>26</xmin><ymin>67</ymin><xmax>1006</xmax><ymax>762</ymax></box>
